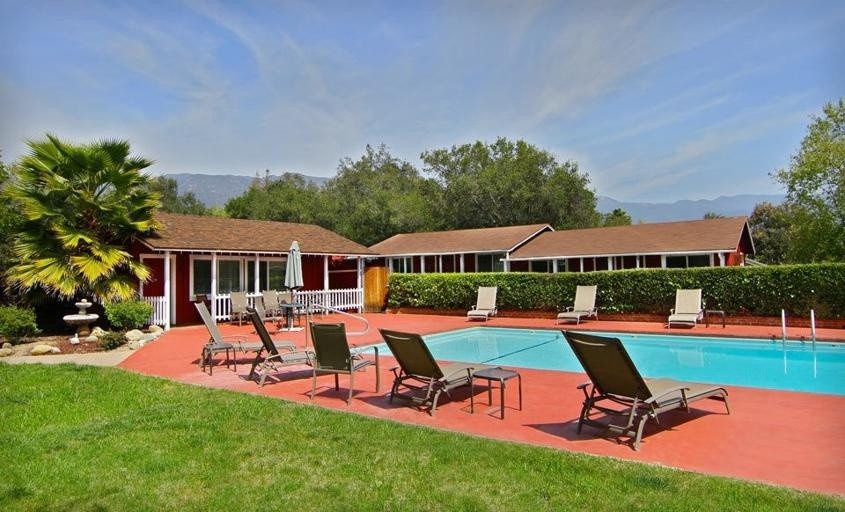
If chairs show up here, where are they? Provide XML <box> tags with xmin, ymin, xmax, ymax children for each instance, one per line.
<box><xmin>240</xmin><ymin>305</ymin><xmax>318</xmax><ymax>387</ymax></box>
<box><xmin>195</xmin><ymin>300</ymin><xmax>297</xmax><ymax>373</ymax></box>
<box><xmin>230</xmin><ymin>291</ymin><xmax>258</xmax><ymax>327</ymax></box>
<box><xmin>465</xmin><ymin>285</ymin><xmax>498</xmax><ymax>322</ymax></box>
<box><xmin>556</xmin><ymin>284</ymin><xmax>601</xmax><ymax>327</ymax></box>
<box><xmin>262</xmin><ymin>288</ymin><xmax>297</xmax><ymax>320</ymax></box>
<box><xmin>278</xmin><ymin>293</ymin><xmax>306</xmax><ymax>320</ymax></box>
<box><xmin>558</xmin><ymin>328</ymin><xmax>734</xmax><ymax>451</ymax></box>
<box><xmin>664</xmin><ymin>289</ymin><xmax>705</xmax><ymax>327</ymax></box>
<box><xmin>306</xmin><ymin>319</ymin><xmax>382</xmax><ymax>405</ymax></box>
<box><xmin>376</xmin><ymin>326</ymin><xmax>489</xmax><ymax>415</ymax></box>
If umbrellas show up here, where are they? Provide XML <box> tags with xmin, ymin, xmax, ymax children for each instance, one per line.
<box><xmin>283</xmin><ymin>241</ymin><xmax>304</xmax><ymax>304</ymax></box>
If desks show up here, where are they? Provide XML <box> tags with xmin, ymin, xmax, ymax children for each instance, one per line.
<box><xmin>278</xmin><ymin>304</ymin><xmax>304</xmax><ymax>332</ymax></box>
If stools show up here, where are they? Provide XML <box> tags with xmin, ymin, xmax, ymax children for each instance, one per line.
<box><xmin>202</xmin><ymin>343</ymin><xmax>238</xmax><ymax>376</ymax></box>
<box><xmin>470</xmin><ymin>368</ymin><xmax>521</xmax><ymax>422</ymax></box>
<box><xmin>705</xmin><ymin>310</ymin><xmax>726</xmax><ymax>328</ymax></box>
